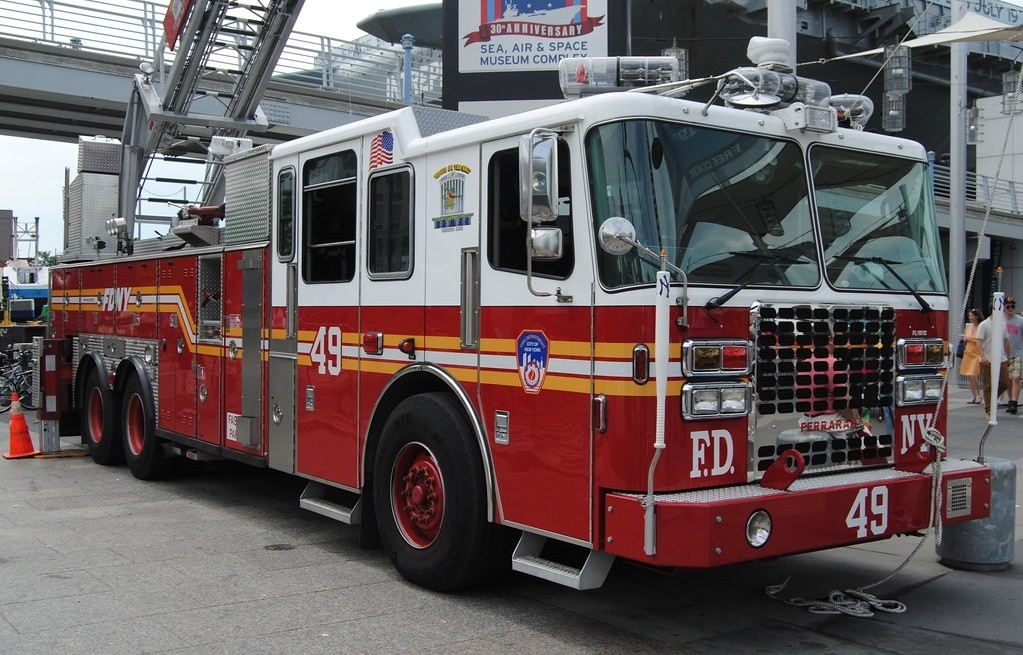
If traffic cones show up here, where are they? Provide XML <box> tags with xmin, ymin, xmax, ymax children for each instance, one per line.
<box><xmin>0</xmin><ymin>392</ymin><xmax>41</xmax><ymax>460</ymax></box>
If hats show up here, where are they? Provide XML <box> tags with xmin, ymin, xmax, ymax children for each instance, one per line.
<box><xmin>1003</xmin><ymin>296</ymin><xmax>1016</xmax><ymax>304</ymax></box>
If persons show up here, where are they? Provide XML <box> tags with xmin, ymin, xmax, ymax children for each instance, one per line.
<box><xmin>959</xmin><ymin>296</ymin><xmax>1023</xmax><ymax>421</ymax></box>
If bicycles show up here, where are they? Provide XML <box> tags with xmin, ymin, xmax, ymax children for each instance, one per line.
<box><xmin>0</xmin><ymin>343</ymin><xmax>39</xmax><ymax>413</ymax></box>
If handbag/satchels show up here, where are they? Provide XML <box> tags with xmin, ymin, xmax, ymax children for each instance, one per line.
<box><xmin>956</xmin><ymin>339</ymin><xmax>966</xmax><ymax>358</ymax></box>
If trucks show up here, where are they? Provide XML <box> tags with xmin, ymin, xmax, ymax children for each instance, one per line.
<box><xmin>0</xmin><ymin>267</ymin><xmax>53</xmax><ymax>367</ymax></box>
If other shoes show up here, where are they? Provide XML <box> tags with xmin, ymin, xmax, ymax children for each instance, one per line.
<box><xmin>1006</xmin><ymin>400</ymin><xmax>1018</xmax><ymax>414</ymax></box>
<box><xmin>985</xmin><ymin>411</ymin><xmax>990</xmax><ymax>420</ymax></box>
<box><xmin>967</xmin><ymin>398</ymin><xmax>976</xmax><ymax>404</ymax></box>
<box><xmin>975</xmin><ymin>396</ymin><xmax>982</xmax><ymax>404</ymax></box>
<box><xmin>997</xmin><ymin>400</ymin><xmax>1004</xmax><ymax>406</ymax></box>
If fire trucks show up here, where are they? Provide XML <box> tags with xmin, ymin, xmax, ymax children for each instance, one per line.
<box><xmin>30</xmin><ymin>0</ymin><xmax>1006</xmax><ymax>596</ymax></box>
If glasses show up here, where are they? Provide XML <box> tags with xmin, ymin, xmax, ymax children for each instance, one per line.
<box><xmin>1004</xmin><ymin>306</ymin><xmax>1015</xmax><ymax>309</ymax></box>
